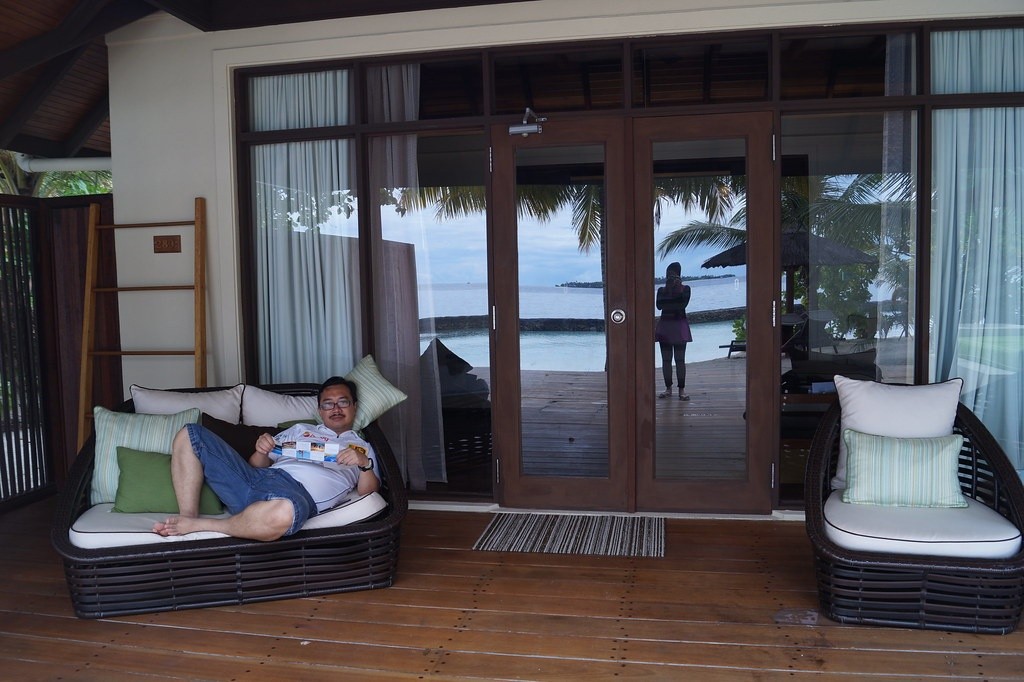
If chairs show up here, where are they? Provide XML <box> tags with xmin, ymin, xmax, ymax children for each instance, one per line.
<box><xmin>804</xmin><ymin>383</ymin><xmax>1024</xmax><ymax>634</ymax></box>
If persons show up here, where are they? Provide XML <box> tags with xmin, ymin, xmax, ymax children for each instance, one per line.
<box><xmin>656</xmin><ymin>262</ymin><xmax>692</xmax><ymax>400</ymax></box>
<box><xmin>153</xmin><ymin>376</ymin><xmax>382</xmax><ymax>542</ymax></box>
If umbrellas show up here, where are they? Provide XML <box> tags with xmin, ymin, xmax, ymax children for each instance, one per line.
<box><xmin>701</xmin><ymin>224</ymin><xmax>879</xmax><ymax>314</ymax></box>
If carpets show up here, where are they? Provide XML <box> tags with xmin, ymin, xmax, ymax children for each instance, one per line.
<box><xmin>471</xmin><ymin>512</ymin><xmax>665</xmax><ymax>559</ymax></box>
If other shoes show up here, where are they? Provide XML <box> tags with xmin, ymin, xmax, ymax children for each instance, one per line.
<box><xmin>679</xmin><ymin>394</ymin><xmax>690</xmax><ymax>401</ymax></box>
<box><xmin>659</xmin><ymin>391</ymin><xmax>672</xmax><ymax>398</ymax></box>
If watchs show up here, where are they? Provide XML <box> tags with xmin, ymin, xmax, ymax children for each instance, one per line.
<box><xmin>358</xmin><ymin>458</ymin><xmax>374</xmax><ymax>472</ymax></box>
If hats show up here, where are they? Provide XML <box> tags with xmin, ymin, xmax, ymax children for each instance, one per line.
<box><xmin>667</xmin><ymin>263</ymin><xmax>681</xmax><ymax>276</ymax></box>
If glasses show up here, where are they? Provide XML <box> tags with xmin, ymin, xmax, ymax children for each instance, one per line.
<box><xmin>319</xmin><ymin>399</ymin><xmax>353</xmax><ymax>411</ymax></box>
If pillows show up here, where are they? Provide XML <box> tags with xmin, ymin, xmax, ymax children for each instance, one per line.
<box><xmin>278</xmin><ymin>419</ymin><xmax>319</xmax><ymax>431</ymax></box>
<box><xmin>240</xmin><ymin>384</ymin><xmax>319</xmax><ymax>428</ymax></box>
<box><xmin>201</xmin><ymin>411</ymin><xmax>288</xmax><ymax>464</ymax></box>
<box><xmin>313</xmin><ymin>354</ymin><xmax>408</xmax><ymax>431</ymax></box>
<box><xmin>831</xmin><ymin>374</ymin><xmax>964</xmax><ymax>489</ymax></box>
<box><xmin>843</xmin><ymin>428</ymin><xmax>971</xmax><ymax>510</ymax></box>
<box><xmin>129</xmin><ymin>383</ymin><xmax>246</xmax><ymax>425</ymax></box>
<box><xmin>91</xmin><ymin>406</ymin><xmax>200</xmax><ymax>505</ymax></box>
<box><xmin>106</xmin><ymin>446</ymin><xmax>225</xmax><ymax>515</ymax></box>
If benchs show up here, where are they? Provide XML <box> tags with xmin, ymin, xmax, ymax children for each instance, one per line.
<box><xmin>50</xmin><ymin>382</ymin><xmax>409</xmax><ymax>620</ymax></box>
